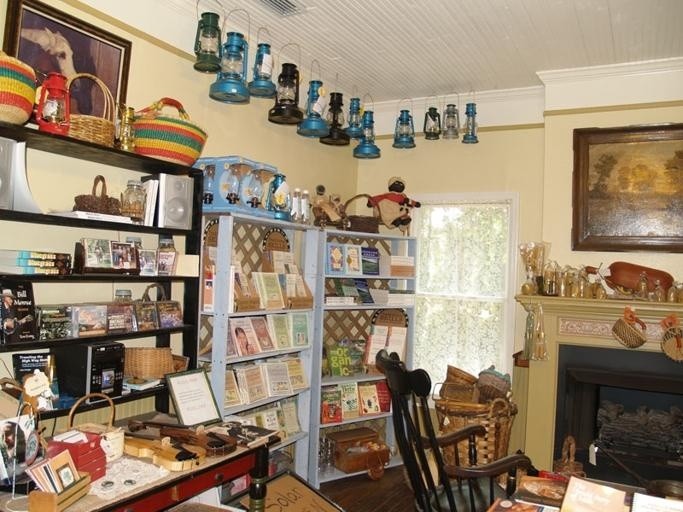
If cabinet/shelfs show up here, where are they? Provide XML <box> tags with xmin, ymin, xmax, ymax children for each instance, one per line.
<box><xmin>295</xmin><ymin>221</ymin><xmax>419</xmax><ymax>486</ymax></box>
<box><xmin>199</xmin><ymin>211</ymin><xmax>325</xmax><ymax>493</ymax></box>
<box><xmin>0</xmin><ymin>119</ymin><xmax>201</xmax><ymax>443</ymax></box>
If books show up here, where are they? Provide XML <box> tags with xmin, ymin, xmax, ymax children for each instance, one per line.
<box><xmin>0</xmin><ymin>414</ymin><xmax>38</xmax><ymax>481</ymax></box>
<box><xmin>0</xmin><ymin>232</ymin><xmax>190</xmax><ymax>338</ymax></box>
<box><xmin>481</xmin><ymin>474</ymin><xmax>683</xmax><ymax>512</ymax></box>
<box><xmin>24</xmin><ymin>429</ymin><xmax>90</xmax><ymax>495</ymax></box>
<box><xmin>218</xmin><ymin>238</ymin><xmax>405</xmax><ymax>422</ymax></box>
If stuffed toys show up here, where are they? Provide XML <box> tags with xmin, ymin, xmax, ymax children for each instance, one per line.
<box><xmin>367</xmin><ymin>175</ymin><xmax>421</xmax><ymax>229</ymax></box>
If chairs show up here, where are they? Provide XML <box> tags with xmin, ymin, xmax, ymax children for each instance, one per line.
<box><xmin>373</xmin><ymin>349</ymin><xmax>535</xmax><ymax>511</ymax></box>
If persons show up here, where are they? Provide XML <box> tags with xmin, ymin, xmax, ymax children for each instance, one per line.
<box><xmin>1</xmin><ymin>423</ymin><xmax>26</xmax><ymax>464</ymax></box>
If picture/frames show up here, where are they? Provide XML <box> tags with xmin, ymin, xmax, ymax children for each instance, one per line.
<box><xmin>220</xmin><ymin>470</ymin><xmax>341</xmax><ymax>511</ymax></box>
<box><xmin>570</xmin><ymin>122</ymin><xmax>683</xmax><ymax>255</ymax></box>
<box><xmin>165</xmin><ymin>365</ymin><xmax>222</xmax><ymax>428</ymax></box>
<box><xmin>20</xmin><ymin>448</ymin><xmax>80</xmax><ymax>493</ymax></box>
<box><xmin>342</xmin><ymin>245</ymin><xmax>363</xmax><ymax>278</ymax></box>
<box><xmin>79</xmin><ymin>236</ymin><xmax>177</xmax><ymax>277</ymax></box>
<box><xmin>325</xmin><ymin>242</ymin><xmax>343</xmax><ymax>276</ymax></box>
<box><xmin>0</xmin><ymin>0</ymin><xmax>133</xmax><ymax>120</ymax></box>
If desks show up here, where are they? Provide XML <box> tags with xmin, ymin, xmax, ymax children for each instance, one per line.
<box><xmin>0</xmin><ymin>408</ymin><xmax>279</xmax><ymax>511</ymax></box>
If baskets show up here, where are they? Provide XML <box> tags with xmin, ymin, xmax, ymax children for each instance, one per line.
<box><xmin>660</xmin><ymin>327</ymin><xmax>682</xmax><ymax>362</ymax></box>
<box><xmin>433</xmin><ymin>401</ymin><xmax>517</xmax><ymax>483</ymax></box>
<box><xmin>478</xmin><ymin>384</ymin><xmax>513</xmax><ymax>404</ymax></box>
<box><xmin>554</xmin><ymin>435</ymin><xmax>584</xmax><ymax>472</ymax></box>
<box><xmin>343</xmin><ymin>194</ymin><xmax>382</xmax><ymax>234</ymax></box>
<box><xmin>446</xmin><ymin>365</ymin><xmax>477</xmax><ymax>385</ymax></box>
<box><xmin>432</xmin><ymin>381</ymin><xmax>475</xmax><ymax>403</ymax></box>
<box><xmin>74</xmin><ymin>176</ymin><xmax>121</xmax><ymax>214</ymax></box>
<box><xmin>478</xmin><ymin>366</ymin><xmax>511</xmax><ymax>393</ymax></box>
<box><xmin>0</xmin><ymin>52</ymin><xmax>36</xmax><ymax>125</ymax></box>
<box><xmin>612</xmin><ymin>317</ymin><xmax>647</xmax><ymax>349</ymax></box>
<box><xmin>65</xmin><ymin>72</ymin><xmax>117</xmax><ymax>148</ymax></box>
<box><xmin>132</xmin><ymin>98</ymin><xmax>208</xmax><ymax>167</ymax></box>
<box><xmin>133</xmin><ymin>284</ymin><xmax>184</xmax><ymax>331</ymax></box>
<box><xmin>125</xmin><ymin>347</ymin><xmax>175</xmax><ymax>381</ymax></box>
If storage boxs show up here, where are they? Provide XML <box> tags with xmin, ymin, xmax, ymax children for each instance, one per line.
<box><xmin>327</xmin><ymin>426</ymin><xmax>390</xmax><ymax>474</ymax></box>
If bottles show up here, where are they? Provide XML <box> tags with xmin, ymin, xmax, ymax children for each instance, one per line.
<box><xmin>633</xmin><ymin>265</ymin><xmax>651</xmax><ymax>304</ymax></box>
<box><xmin>118</xmin><ymin>177</ymin><xmax>147</xmax><ymax>221</ymax></box>
<box><xmin>290</xmin><ymin>188</ymin><xmax>302</xmax><ymax>223</ymax></box>
<box><xmin>653</xmin><ymin>277</ymin><xmax>665</xmax><ymax>304</ymax></box>
<box><xmin>302</xmin><ymin>189</ymin><xmax>312</xmax><ymax>225</ymax></box>
<box><xmin>543</xmin><ymin>258</ymin><xmax>607</xmax><ymax>299</ymax></box>
<box><xmin>112</xmin><ymin>288</ymin><xmax>137</xmax><ymax>332</ymax></box>
<box><xmin>666</xmin><ymin>280</ymin><xmax>680</xmax><ymax>305</ymax></box>
